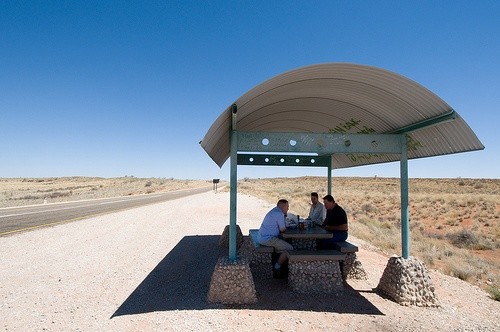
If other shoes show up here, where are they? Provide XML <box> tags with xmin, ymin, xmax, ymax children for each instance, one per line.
<box><xmin>274</xmin><ymin>263</ymin><xmax>281</xmax><ymax>269</ymax></box>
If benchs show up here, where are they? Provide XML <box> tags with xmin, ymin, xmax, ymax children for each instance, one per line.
<box><xmin>247</xmin><ymin>229</ymin><xmax>274</xmax><ymax>279</ymax></box>
<box><xmin>285</xmin><ymin>250</ymin><xmax>346</xmax><ymax>294</ymax></box>
<box><xmin>333</xmin><ymin>238</ymin><xmax>369</xmax><ymax>280</ymax></box>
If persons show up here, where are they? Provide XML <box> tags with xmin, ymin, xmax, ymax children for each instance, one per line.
<box><xmin>305</xmin><ymin>192</ymin><xmax>326</xmax><ymax>226</ymax></box>
<box><xmin>316</xmin><ymin>195</ymin><xmax>348</xmax><ymax>250</ymax></box>
<box><xmin>258</xmin><ymin>199</ymin><xmax>293</xmax><ymax>272</ymax></box>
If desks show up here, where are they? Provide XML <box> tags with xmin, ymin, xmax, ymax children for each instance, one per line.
<box><xmin>280</xmin><ymin>226</ymin><xmax>332</xmax><ymax>249</ymax></box>
